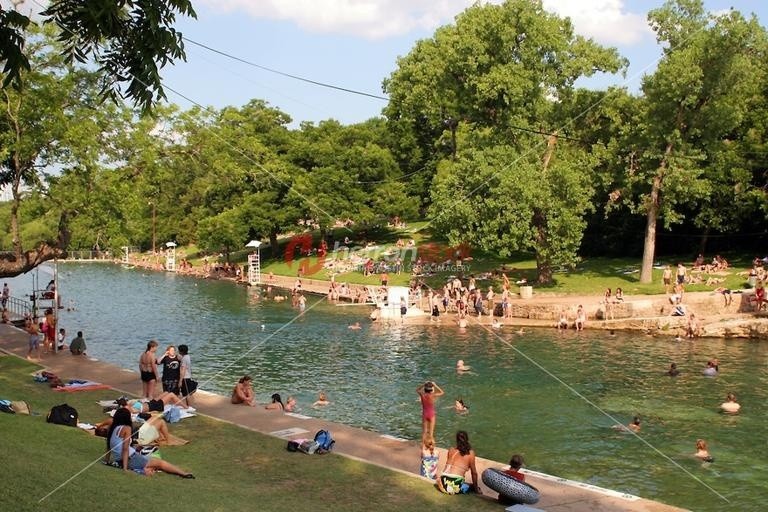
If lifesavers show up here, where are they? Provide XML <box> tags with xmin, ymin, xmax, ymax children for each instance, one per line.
<box><xmin>482</xmin><ymin>467</ymin><xmax>540</xmax><ymax>505</ymax></box>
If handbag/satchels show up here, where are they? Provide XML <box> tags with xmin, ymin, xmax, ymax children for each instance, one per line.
<box><xmin>314</xmin><ymin>429</ymin><xmax>336</xmax><ymax>454</ymax></box>
<box><xmin>46</xmin><ymin>403</ymin><xmax>78</xmax><ymax>427</ymax></box>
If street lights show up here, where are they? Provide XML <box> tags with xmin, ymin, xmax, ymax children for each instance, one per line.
<box><xmin>148</xmin><ymin>201</ymin><xmax>156</xmax><ymax>254</ymax></box>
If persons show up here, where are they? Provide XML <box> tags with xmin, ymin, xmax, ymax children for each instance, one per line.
<box><xmin>230</xmin><ymin>374</ymin><xmax>256</xmax><ymax>405</ymax></box>
<box><xmin>627</xmin><ymin>415</ymin><xmax>640</xmax><ymax>431</ymax></box>
<box><xmin>694</xmin><ymin>437</ymin><xmax>709</xmax><ymax>458</ymax></box>
<box><xmin>264</xmin><ymin>391</ymin><xmax>284</xmax><ymax>410</ymax></box>
<box><xmin>312</xmin><ymin>391</ymin><xmax>330</xmax><ymax>406</ymax></box>
<box><xmin>703</xmin><ymin>357</ymin><xmax>719</xmax><ymax>375</ymax></box>
<box><xmin>438</xmin><ymin>429</ymin><xmax>482</xmax><ymax>495</ymax></box>
<box><xmin>720</xmin><ymin>391</ymin><xmax>742</xmax><ymax>412</ymax></box>
<box><xmin>1</xmin><ymin>277</ymin><xmax>196</xmax><ymax>480</ymax></box>
<box><xmin>415</xmin><ymin>380</ymin><xmax>446</xmax><ymax>454</ymax></box>
<box><xmin>131</xmin><ymin>212</ymin><xmax>767</xmax><ymax>337</ymax></box>
<box><xmin>441</xmin><ymin>398</ymin><xmax>468</xmax><ymax>415</ymax></box>
<box><xmin>666</xmin><ymin>361</ymin><xmax>681</xmax><ymax>377</ymax></box>
<box><xmin>498</xmin><ymin>454</ymin><xmax>525</xmax><ymax>506</ymax></box>
<box><xmin>446</xmin><ymin>360</ymin><xmax>466</xmax><ymax>371</ymax></box>
<box><xmin>282</xmin><ymin>395</ymin><xmax>297</xmax><ymax>412</ymax></box>
